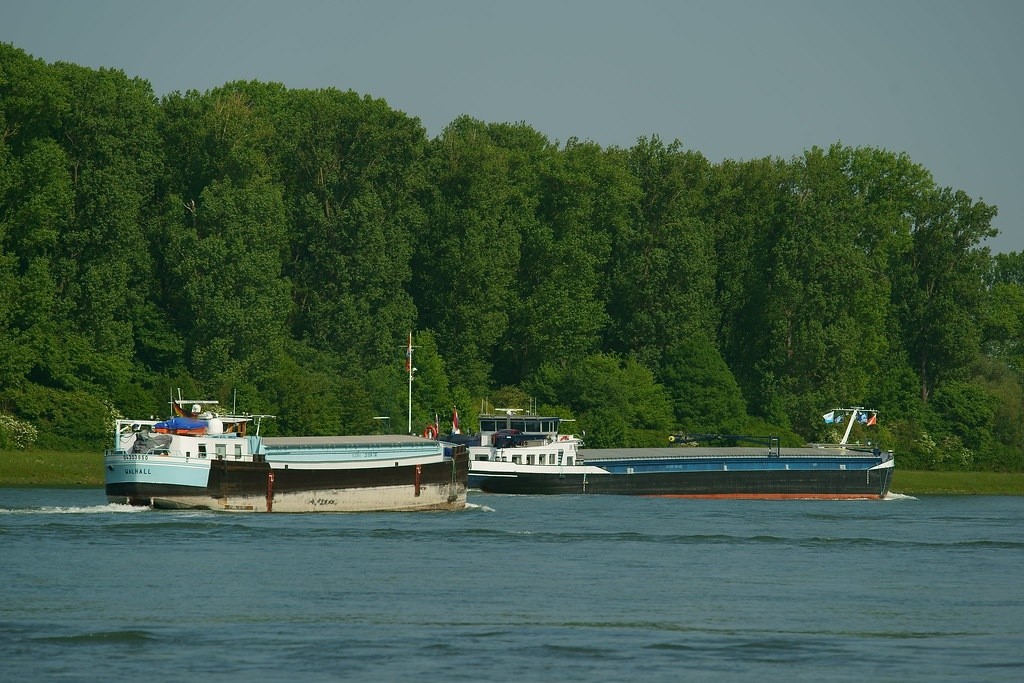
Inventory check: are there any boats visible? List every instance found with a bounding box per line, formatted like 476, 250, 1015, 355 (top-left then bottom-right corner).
424, 408, 896, 500
104, 390, 472, 513
464, 396, 611, 494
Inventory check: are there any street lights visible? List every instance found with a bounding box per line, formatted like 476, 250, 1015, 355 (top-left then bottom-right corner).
409, 368, 418, 432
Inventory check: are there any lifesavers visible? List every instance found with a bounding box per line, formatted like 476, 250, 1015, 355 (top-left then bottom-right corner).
560, 436, 569, 441
424, 426, 437, 439
